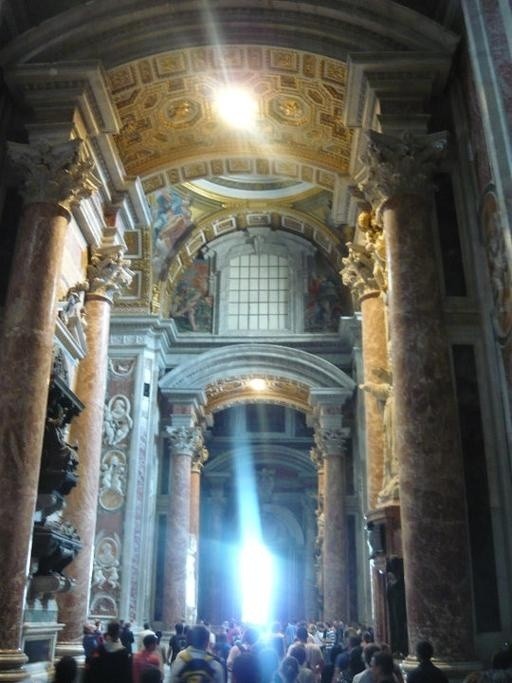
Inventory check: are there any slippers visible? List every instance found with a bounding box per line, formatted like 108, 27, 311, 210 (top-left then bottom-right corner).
177, 649, 216, 682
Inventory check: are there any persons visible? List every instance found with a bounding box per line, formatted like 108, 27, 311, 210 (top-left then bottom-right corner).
90, 394, 136, 590
